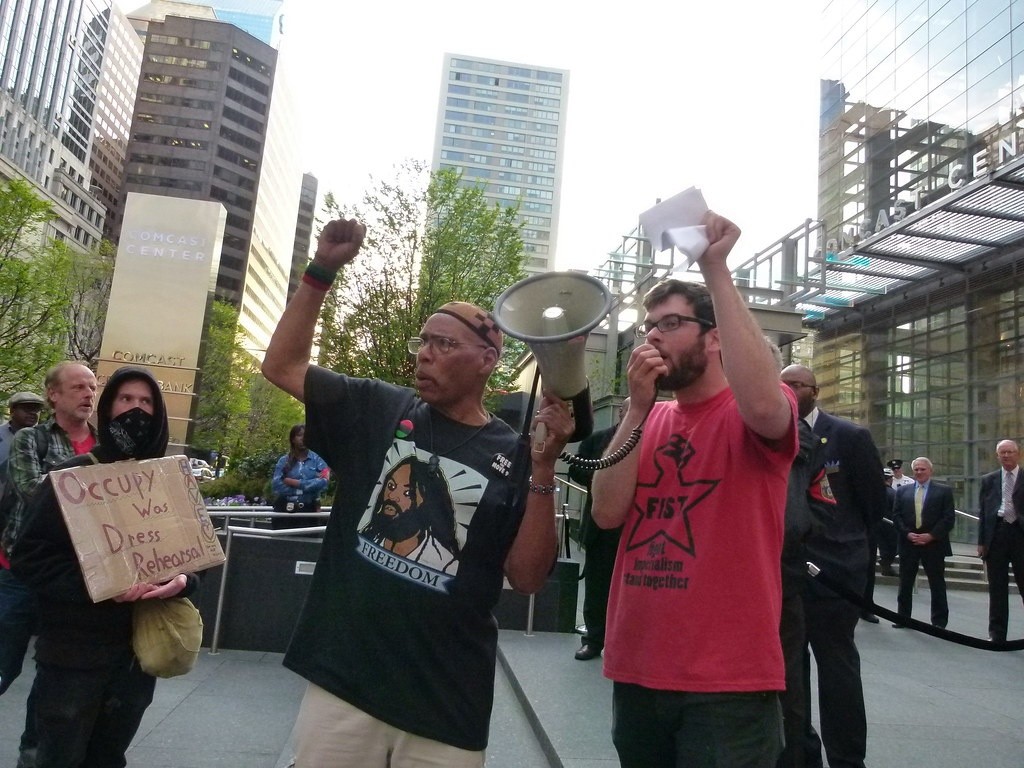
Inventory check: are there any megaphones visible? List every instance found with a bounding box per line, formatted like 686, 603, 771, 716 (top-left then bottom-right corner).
493, 270, 612, 444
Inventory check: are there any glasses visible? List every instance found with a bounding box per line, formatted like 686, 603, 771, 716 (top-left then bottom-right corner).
632, 313, 716, 339
782, 380, 815, 391
406, 336, 487, 355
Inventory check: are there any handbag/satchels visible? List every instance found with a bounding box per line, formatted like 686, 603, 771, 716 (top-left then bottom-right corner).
128, 593, 205, 680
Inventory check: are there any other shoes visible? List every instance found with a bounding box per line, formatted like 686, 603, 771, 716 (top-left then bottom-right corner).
881, 565, 900, 577
933, 624, 945, 635
861, 613, 879, 623
893, 617, 909, 628
989, 637, 1006, 650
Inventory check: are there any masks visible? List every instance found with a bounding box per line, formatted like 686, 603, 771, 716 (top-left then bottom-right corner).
106, 407, 157, 460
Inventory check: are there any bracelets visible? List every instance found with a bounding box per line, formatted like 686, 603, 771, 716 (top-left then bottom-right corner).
528, 475, 555, 495
302, 260, 337, 290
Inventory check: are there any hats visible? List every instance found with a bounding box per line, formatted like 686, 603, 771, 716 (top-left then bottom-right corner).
8, 391, 45, 408
883, 467, 895, 476
887, 459, 903, 469
433, 301, 503, 356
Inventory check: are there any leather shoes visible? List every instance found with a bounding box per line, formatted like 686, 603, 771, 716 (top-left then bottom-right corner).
575, 644, 601, 660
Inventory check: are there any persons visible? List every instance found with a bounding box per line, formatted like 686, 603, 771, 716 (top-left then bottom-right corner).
587, 211, 800, 768
567, 395, 632, 663
878, 459, 917, 577
257, 219, 576, 768
269, 422, 331, 540
890, 458, 956, 630
15, 365, 202, 768
0, 360, 101, 698
761, 363, 886, 768
976, 439, 1024, 644
858, 546, 881, 624
0, 392, 45, 535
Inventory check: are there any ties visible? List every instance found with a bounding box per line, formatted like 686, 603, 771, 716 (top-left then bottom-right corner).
1003, 471, 1018, 525
915, 483, 923, 529
